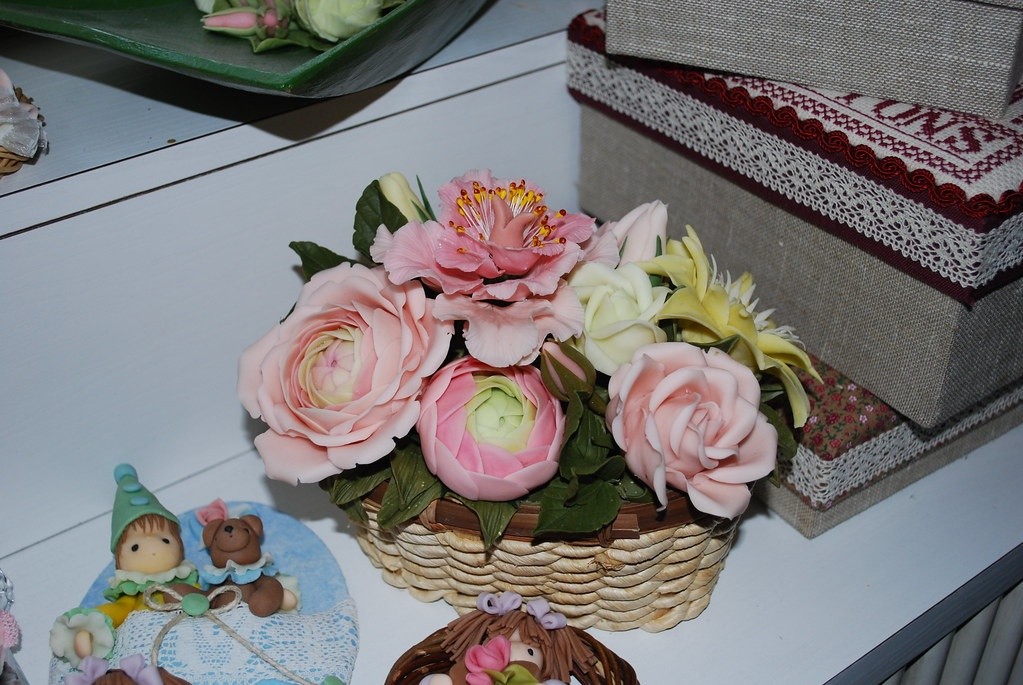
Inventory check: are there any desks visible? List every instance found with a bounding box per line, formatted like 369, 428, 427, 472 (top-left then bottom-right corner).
0, 0, 1023, 685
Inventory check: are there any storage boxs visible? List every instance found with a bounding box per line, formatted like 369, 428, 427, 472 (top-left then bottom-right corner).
751, 387, 1020, 539
563, 8, 1022, 427
605, 0, 1023, 115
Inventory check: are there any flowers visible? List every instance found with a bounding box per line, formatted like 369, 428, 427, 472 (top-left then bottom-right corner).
237, 170, 821, 543
194, 0, 407, 53
